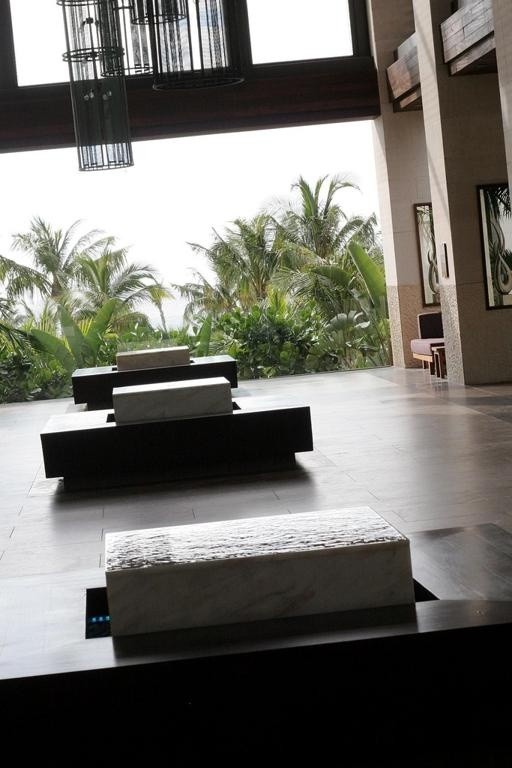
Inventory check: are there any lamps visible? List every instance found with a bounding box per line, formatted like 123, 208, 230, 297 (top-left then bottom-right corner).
58, 1, 247, 172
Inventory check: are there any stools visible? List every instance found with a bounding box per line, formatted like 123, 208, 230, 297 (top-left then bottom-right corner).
432, 345, 448, 380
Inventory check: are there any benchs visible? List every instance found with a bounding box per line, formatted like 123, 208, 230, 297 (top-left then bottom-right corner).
1, 502, 512, 768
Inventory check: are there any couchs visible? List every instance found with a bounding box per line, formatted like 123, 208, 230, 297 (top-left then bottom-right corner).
411, 311, 445, 375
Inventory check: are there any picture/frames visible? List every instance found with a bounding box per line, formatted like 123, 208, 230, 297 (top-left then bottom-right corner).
413, 202, 442, 307
475, 182, 512, 311
